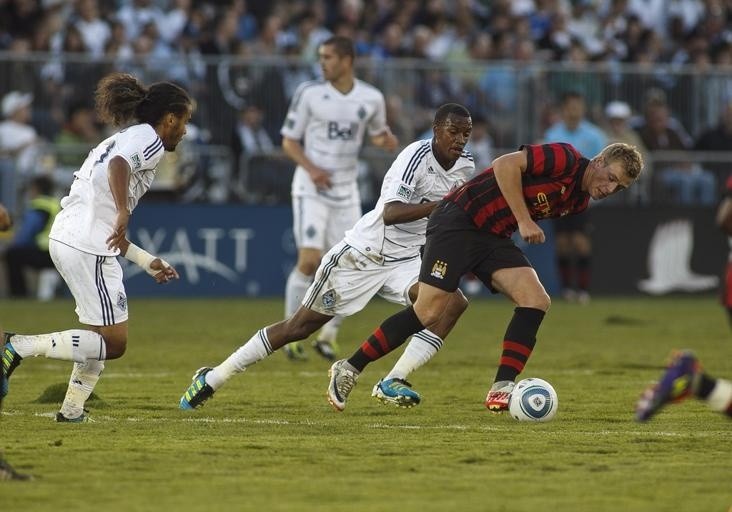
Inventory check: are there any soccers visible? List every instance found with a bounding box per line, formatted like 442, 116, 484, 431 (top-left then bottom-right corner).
508, 377, 558, 422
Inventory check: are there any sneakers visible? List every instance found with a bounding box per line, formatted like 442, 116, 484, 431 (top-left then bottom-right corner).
371, 376, 422, 409
179, 365, 217, 410
311, 332, 341, 360
0, 331, 24, 399
632, 349, 705, 423
56, 411, 102, 424
326, 359, 357, 412
285, 339, 309, 360
483, 379, 517, 412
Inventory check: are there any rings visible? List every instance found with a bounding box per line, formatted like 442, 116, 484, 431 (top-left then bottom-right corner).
115, 231, 119, 236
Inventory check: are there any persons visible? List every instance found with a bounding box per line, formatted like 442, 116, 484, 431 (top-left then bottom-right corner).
709, 164, 731, 316
1, 0, 731, 209
326, 140, 645, 413
3, 174, 64, 298
632, 341, 731, 430
279, 36, 399, 363
177, 101, 475, 411
1, 73, 201, 423
550, 208, 598, 306
